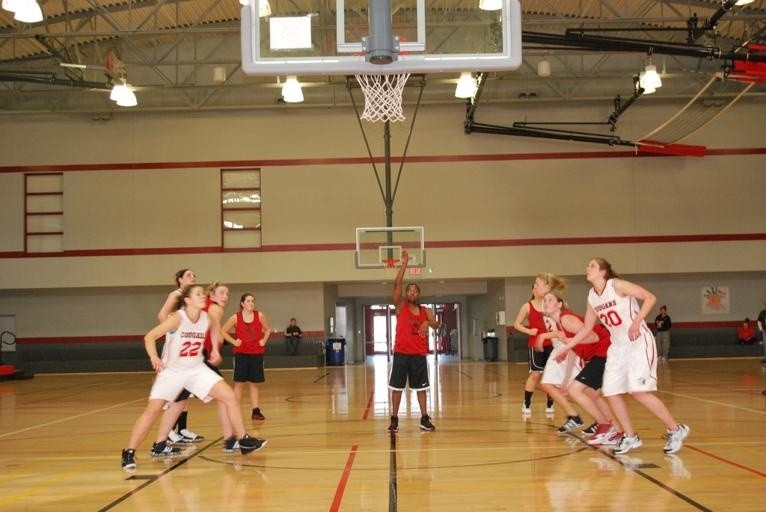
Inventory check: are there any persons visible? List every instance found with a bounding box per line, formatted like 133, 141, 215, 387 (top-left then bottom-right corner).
736, 317, 760, 346
120, 284, 269, 469
221, 293, 272, 421
653, 304, 672, 362
534, 271, 602, 435
514, 281, 556, 415
386, 249, 440, 435
536, 292, 627, 446
151, 279, 243, 457
283, 317, 303, 355
758, 307, 766, 367
160, 268, 207, 442
552, 256, 692, 456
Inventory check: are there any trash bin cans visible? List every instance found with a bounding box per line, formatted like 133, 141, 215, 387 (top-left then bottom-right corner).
482, 337, 498, 362
327, 338, 346, 366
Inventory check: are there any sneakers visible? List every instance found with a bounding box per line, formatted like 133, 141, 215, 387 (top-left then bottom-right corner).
545, 403, 554, 413
238, 433, 267, 457
251, 406, 266, 421
556, 415, 584, 435
178, 428, 206, 443
612, 432, 643, 455
387, 414, 400, 433
420, 416, 435, 432
167, 430, 184, 444
150, 440, 183, 457
120, 448, 137, 469
223, 433, 240, 453
521, 403, 531, 414
580, 422, 625, 446
663, 423, 690, 455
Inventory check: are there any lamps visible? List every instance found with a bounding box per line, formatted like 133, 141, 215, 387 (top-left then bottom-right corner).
0, 0, 43, 24
239, 0, 273, 19
477, 0, 504, 11
281, 77, 305, 103
635, 50, 662, 95
454, 68, 478, 98
108, 60, 137, 107
721, 0, 755, 6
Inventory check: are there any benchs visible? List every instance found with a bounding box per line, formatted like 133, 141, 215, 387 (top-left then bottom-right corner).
1, 337, 325, 374
505, 321, 765, 364
